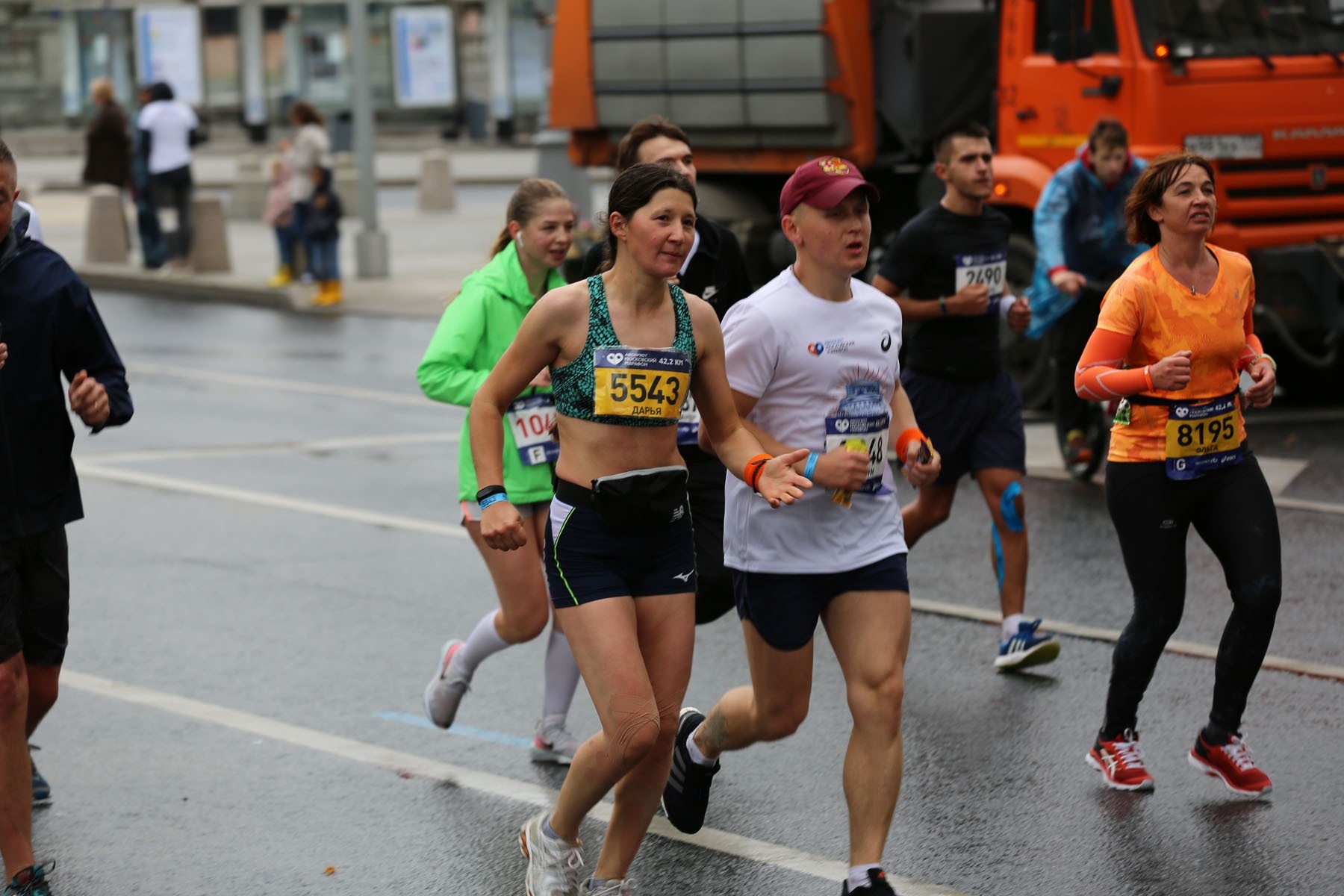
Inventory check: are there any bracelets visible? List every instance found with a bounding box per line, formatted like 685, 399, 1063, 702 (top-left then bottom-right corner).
939, 295, 950, 318
1248, 353, 1278, 371
479, 493, 508, 509
744, 453, 777, 493
999, 294, 1014, 320
1144, 363, 1155, 393
799, 450, 823, 481
895, 427, 927, 463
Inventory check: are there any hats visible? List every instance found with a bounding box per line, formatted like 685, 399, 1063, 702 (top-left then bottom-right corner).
778, 156, 881, 219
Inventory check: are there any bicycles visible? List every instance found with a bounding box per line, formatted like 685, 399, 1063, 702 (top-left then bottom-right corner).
1048, 278, 1116, 485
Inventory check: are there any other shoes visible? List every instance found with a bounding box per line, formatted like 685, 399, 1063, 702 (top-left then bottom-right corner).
1064, 429, 1091, 475
268, 263, 290, 286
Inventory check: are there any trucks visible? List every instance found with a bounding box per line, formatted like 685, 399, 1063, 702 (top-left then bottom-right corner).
547, 1, 1344, 418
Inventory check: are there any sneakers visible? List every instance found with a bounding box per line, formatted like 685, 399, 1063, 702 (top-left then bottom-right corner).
530, 724, 584, 764
1084, 726, 1155, 791
518, 808, 637, 896
660, 707, 721, 835
992, 616, 1061, 670
842, 867, 897, 896
1188, 726, 1273, 795
424, 638, 474, 730
27, 744, 53, 805
0, 858, 56, 896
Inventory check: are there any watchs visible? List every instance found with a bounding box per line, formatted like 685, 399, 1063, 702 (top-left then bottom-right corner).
476, 484, 507, 503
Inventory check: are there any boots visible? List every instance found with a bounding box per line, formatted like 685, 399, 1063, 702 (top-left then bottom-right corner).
312, 279, 343, 305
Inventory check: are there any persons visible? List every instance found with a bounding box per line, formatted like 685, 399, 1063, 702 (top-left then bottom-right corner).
418, 177, 594, 769
0, 138, 135, 896
261, 101, 347, 306
582, 114, 947, 623
469, 163, 813, 895
1074, 155, 1282, 798
663, 153, 941, 896
1019, 119, 1153, 487
874, 120, 1063, 672
81, 78, 210, 265
